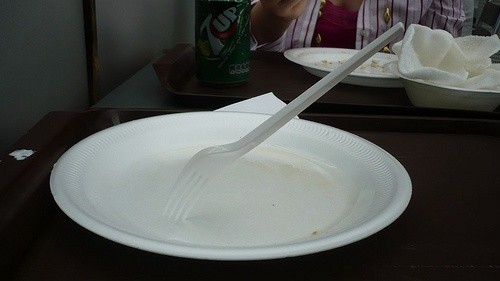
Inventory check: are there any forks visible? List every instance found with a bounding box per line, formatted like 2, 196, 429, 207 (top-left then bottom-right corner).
160, 22, 404, 222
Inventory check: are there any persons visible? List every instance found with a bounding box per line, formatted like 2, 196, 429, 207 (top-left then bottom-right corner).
250, 0, 466, 55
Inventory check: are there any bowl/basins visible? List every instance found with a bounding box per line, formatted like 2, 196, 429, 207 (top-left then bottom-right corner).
382, 61, 500, 110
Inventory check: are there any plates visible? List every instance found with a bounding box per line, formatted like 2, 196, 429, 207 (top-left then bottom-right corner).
50, 111, 412, 261
283, 47, 407, 87
